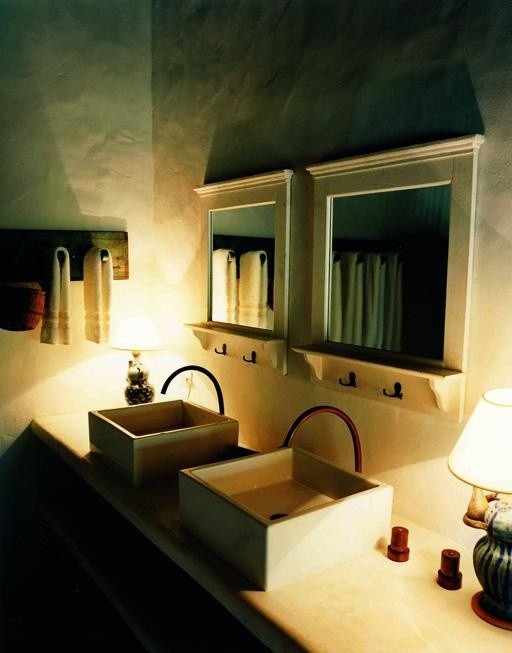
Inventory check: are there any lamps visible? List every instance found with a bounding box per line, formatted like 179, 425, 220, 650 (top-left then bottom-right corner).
445, 388, 512, 631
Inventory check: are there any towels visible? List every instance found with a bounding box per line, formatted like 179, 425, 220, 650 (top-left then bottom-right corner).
40, 243, 115, 346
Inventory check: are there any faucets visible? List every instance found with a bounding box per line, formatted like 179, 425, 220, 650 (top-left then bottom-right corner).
283, 405, 362, 472
160, 364, 224, 414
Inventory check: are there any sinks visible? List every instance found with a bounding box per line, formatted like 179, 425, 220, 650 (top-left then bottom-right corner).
178, 445, 394, 592
88, 399, 240, 488
